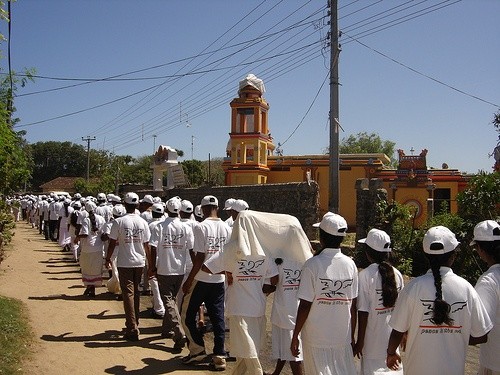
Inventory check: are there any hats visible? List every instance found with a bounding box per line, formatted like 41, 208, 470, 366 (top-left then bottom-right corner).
422, 226, 461, 254
357, 228, 392, 253
6, 192, 250, 215
312, 212, 348, 237
469, 220, 500, 246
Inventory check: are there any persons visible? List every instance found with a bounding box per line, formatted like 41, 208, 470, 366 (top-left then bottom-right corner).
202, 210, 314, 375
290, 213, 359, 375
0, 192, 73, 252
469, 219, 500, 375
387, 227, 493, 375
71, 193, 251, 371
355, 229, 408, 375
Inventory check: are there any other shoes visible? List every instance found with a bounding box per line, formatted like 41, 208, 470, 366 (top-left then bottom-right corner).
83, 288, 96, 297
183, 350, 207, 364
123, 332, 139, 342
161, 330, 175, 338
208, 354, 227, 370
146, 307, 164, 318
171, 334, 187, 354
